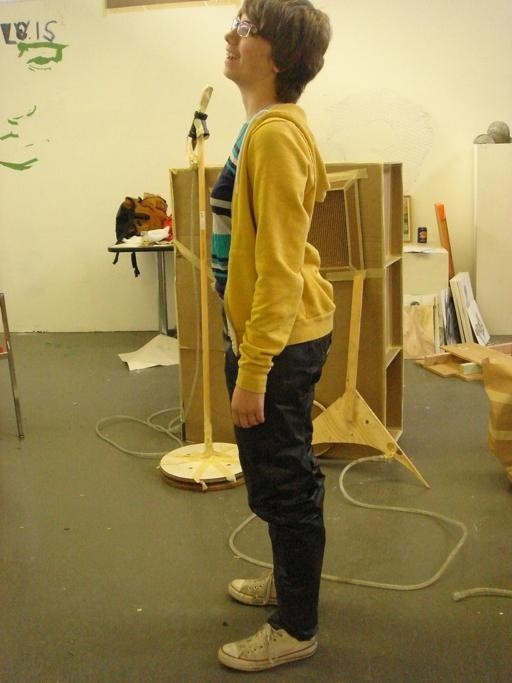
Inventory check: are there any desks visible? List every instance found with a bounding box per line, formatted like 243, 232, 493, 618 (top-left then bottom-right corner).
107, 240, 176, 336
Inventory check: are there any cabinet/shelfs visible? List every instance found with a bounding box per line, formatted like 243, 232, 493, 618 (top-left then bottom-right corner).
169, 159, 406, 460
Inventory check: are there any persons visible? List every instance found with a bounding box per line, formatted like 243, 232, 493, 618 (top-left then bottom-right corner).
210, 0, 373, 671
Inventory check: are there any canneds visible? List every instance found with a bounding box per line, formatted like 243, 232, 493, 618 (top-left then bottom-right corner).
418, 227, 427, 243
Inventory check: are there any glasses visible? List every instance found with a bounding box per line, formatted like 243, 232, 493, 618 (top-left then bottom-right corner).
231, 16, 257, 39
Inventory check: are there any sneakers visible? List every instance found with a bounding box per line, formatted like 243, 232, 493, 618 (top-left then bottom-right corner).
217, 621, 319, 674
228, 572, 280, 608
